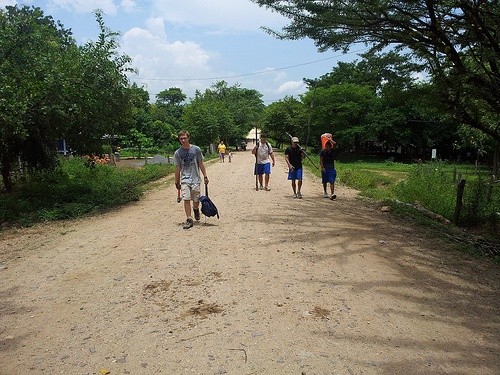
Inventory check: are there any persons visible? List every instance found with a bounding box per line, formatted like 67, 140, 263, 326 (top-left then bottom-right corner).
252, 134, 275, 191
318, 141, 339, 200
217, 140, 233, 163
174, 131, 209, 229
285, 137, 305, 199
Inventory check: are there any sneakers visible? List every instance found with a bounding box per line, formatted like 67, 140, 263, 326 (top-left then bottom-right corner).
193, 208, 200, 220
324, 193, 328, 198
183, 218, 193, 229
265, 185, 271, 191
293, 193, 302, 199
330, 194, 336, 200
260, 185, 263, 189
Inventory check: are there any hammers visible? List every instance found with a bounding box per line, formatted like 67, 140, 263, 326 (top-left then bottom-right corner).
177, 181, 182, 203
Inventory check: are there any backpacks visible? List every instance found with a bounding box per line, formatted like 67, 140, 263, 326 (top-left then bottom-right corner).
199, 183, 219, 219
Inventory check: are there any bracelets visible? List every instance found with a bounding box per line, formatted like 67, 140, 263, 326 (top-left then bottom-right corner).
204, 176, 207, 179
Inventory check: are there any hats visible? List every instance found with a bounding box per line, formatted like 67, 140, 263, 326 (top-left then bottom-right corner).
292, 137, 299, 143
